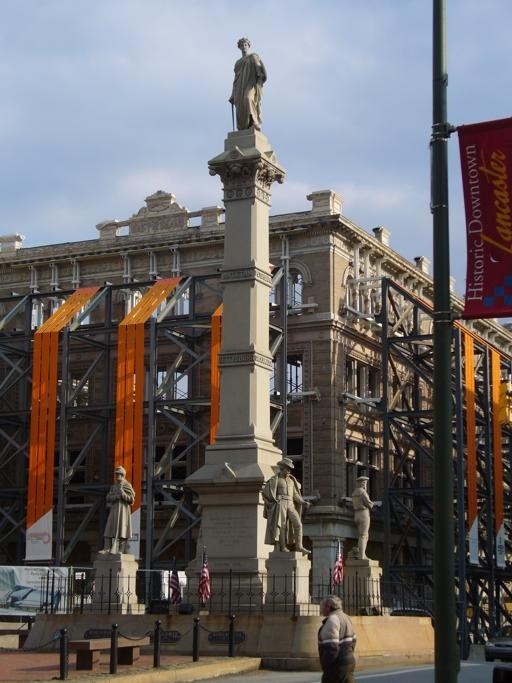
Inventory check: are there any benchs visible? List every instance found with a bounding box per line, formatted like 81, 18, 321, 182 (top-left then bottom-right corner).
68, 635, 151, 671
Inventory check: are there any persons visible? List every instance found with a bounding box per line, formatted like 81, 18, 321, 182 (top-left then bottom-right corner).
98, 466, 135, 554
317, 595, 356, 683
229, 38, 267, 131
352, 476, 374, 560
262, 458, 312, 555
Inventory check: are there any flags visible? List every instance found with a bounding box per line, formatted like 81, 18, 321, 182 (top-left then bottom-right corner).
199, 559, 215, 607
331, 542, 344, 590
168, 559, 182, 604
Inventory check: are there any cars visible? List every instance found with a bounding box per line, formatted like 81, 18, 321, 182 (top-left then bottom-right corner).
388, 607, 433, 616
483, 625, 512, 662
